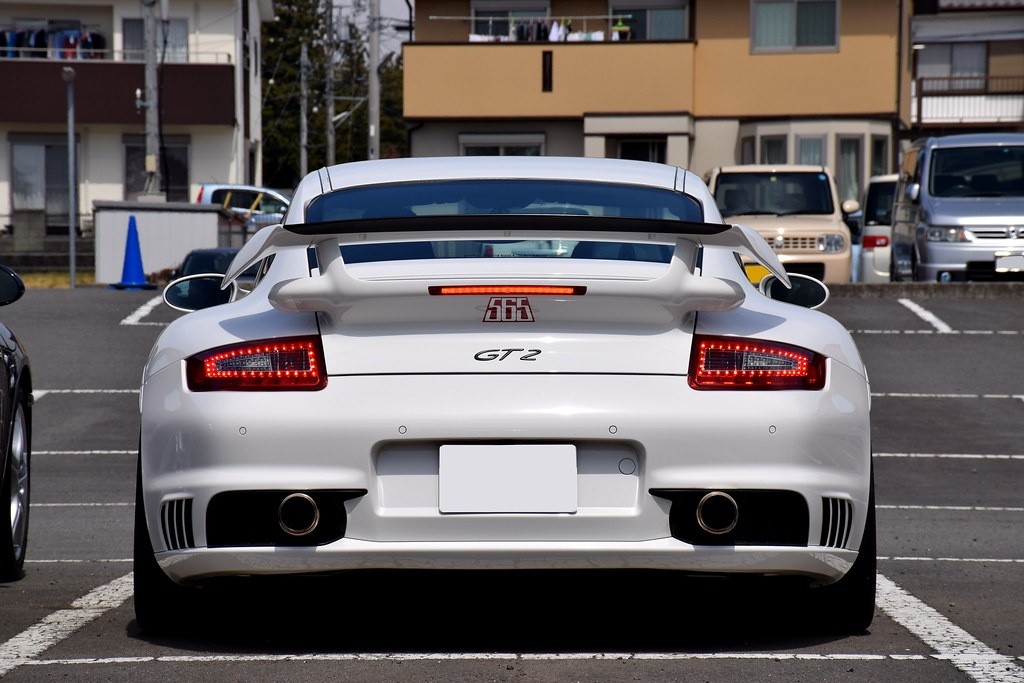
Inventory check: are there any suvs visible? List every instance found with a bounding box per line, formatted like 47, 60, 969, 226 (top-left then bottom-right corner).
707, 164, 860, 286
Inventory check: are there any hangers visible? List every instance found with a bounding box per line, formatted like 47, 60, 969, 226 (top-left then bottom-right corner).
512, 15, 573, 26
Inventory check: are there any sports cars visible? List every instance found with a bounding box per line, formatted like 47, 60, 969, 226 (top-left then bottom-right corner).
134, 154, 875, 635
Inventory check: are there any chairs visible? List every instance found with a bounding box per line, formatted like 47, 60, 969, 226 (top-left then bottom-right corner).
570, 241, 670, 262
938, 165, 970, 191
351, 206, 436, 261
724, 189, 752, 212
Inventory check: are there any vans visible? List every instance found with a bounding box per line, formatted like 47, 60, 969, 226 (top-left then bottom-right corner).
861, 133, 1024, 282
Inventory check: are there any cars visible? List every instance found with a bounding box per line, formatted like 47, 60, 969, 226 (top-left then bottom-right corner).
197, 180, 293, 227
